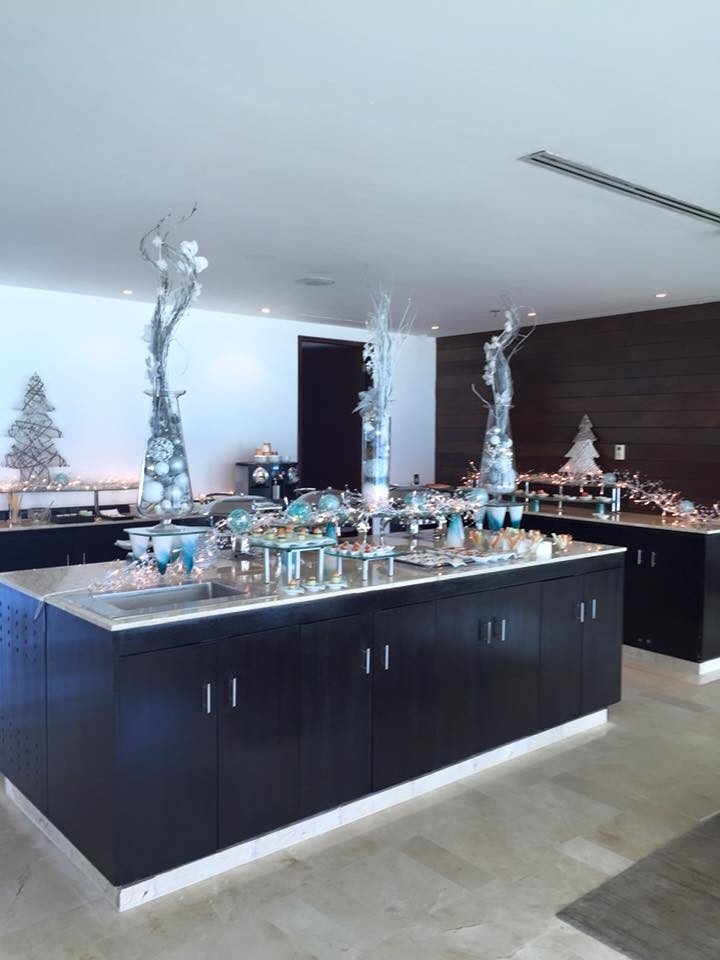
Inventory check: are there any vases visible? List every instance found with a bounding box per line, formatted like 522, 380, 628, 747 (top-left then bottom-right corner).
361, 416, 391, 501
480, 405, 516, 492
136, 389, 194, 518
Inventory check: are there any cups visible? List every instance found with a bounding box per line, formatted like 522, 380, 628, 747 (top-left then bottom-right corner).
470, 529, 540, 552
256, 443, 278, 455
553, 535, 568, 554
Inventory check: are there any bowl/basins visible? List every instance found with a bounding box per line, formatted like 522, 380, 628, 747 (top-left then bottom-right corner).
254, 455, 290, 463
27, 507, 52, 525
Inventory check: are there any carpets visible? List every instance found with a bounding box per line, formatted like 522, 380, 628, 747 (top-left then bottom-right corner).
556, 813, 720, 960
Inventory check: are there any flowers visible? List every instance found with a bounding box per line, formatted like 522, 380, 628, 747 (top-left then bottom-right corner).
138, 203, 209, 389
351, 281, 424, 415
474, 294, 538, 404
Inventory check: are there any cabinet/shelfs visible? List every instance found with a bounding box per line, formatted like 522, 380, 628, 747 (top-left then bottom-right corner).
0, 461, 720, 913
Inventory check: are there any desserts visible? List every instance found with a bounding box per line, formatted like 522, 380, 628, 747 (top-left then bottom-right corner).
332, 541, 379, 553
288, 572, 341, 588
253, 528, 323, 543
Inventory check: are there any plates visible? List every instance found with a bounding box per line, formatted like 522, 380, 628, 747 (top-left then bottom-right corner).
592, 513, 610, 519
281, 579, 348, 594
